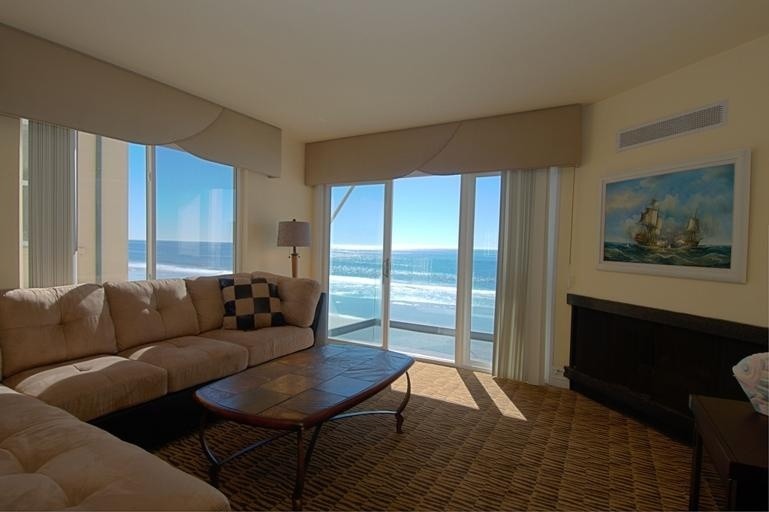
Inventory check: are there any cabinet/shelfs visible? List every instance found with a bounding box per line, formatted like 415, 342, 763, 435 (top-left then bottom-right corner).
559, 292, 768, 449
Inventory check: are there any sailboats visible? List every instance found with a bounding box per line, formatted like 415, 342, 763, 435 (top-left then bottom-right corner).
628, 196, 703, 256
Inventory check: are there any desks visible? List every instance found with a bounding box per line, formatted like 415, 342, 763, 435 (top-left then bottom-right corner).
192, 343, 415, 512
688, 392, 768, 512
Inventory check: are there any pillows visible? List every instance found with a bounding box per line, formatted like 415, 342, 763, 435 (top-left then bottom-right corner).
218, 277, 287, 332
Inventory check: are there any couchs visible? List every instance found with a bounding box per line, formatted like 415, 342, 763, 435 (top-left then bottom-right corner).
0, 381, 231, 512
0, 271, 327, 452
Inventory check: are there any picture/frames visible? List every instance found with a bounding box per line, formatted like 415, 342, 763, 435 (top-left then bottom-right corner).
594, 148, 753, 285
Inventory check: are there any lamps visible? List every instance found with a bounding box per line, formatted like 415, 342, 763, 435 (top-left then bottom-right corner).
276, 219, 311, 279
